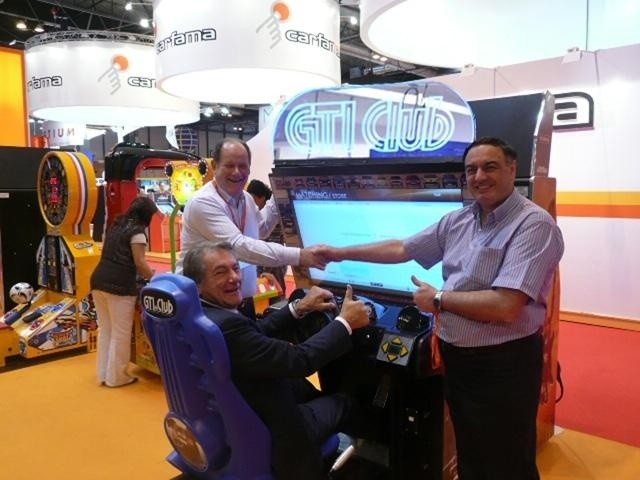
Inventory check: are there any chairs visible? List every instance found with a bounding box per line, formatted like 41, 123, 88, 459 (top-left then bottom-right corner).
140, 272, 363, 479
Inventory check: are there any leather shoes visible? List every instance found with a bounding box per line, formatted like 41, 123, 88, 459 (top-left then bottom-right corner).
128, 377, 138, 384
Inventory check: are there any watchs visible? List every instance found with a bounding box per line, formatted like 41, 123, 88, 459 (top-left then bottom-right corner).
433, 290, 446, 314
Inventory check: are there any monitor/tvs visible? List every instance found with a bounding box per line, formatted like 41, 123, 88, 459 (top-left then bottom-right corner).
269, 172, 530, 304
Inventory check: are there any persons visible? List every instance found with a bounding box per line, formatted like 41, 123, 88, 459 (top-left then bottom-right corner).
181, 241, 393, 477
88, 196, 157, 389
311, 136, 565, 480
247, 178, 272, 210
174, 138, 328, 322
